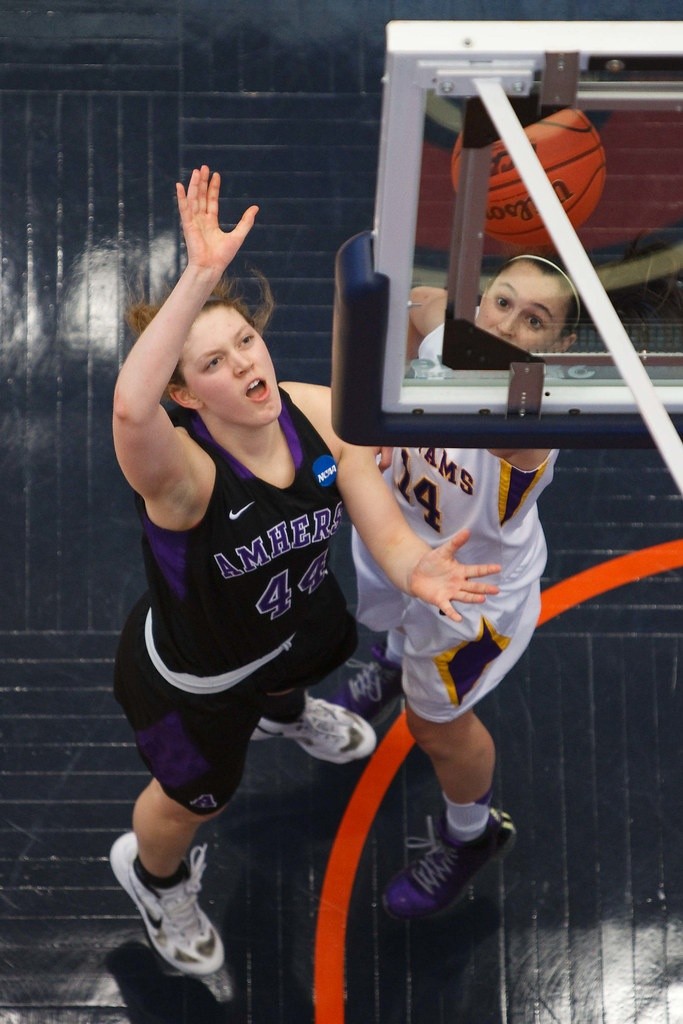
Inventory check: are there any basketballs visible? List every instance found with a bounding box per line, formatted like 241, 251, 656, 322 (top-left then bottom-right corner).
452, 103, 607, 244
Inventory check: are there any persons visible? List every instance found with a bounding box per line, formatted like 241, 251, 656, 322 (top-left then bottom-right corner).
333, 250, 585, 926
109, 162, 503, 976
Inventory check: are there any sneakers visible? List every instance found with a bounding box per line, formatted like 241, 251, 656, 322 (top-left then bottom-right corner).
383, 809, 517, 923
249, 688, 377, 764
328, 645, 403, 724
110, 830, 224, 975
